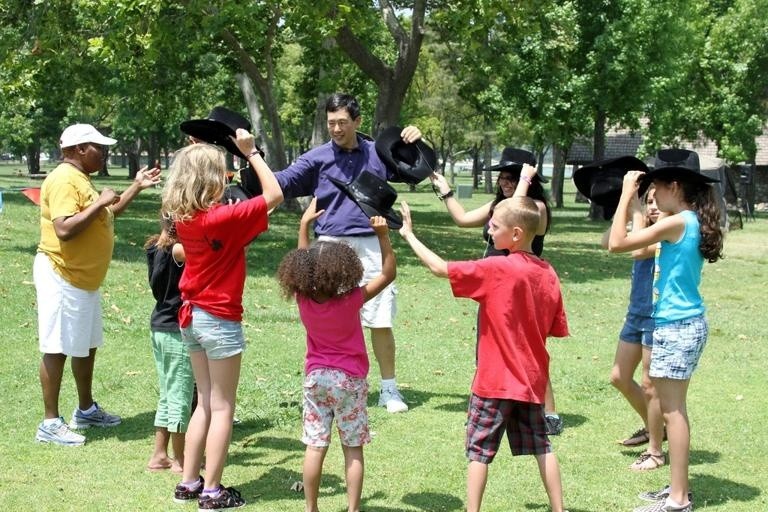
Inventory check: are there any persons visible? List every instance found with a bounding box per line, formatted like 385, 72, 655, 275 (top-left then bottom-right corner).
606, 147, 726, 512
600, 154, 667, 472
399, 195, 570, 512
430, 145, 564, 437
143, 205, 208, 476
31, 123, 164, 449
271, 193, 398, 512
164, 125, 285, 512
265, 90, 424, 415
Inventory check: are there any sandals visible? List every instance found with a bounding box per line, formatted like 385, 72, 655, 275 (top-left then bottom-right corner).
625, 428, 667, 446
630, 452, 666, 470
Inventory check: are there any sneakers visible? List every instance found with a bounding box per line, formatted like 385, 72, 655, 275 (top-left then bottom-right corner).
69, 407, 122, 428
545, 415, 560, 436
37, 420, 86, 446
638, 486, 692, 503
198, 488, 246, 511
634, 500, 693, 512
378, 390, 409, 413
174, 479, 224, 500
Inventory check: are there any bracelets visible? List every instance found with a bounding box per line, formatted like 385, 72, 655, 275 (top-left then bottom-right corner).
246, 150, 261, 158
520, 174, 532, 186
437, 190, 453, 202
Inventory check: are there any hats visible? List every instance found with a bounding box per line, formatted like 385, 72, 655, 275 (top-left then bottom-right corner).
325, 172, 403, 230
640, 149, 723, 185
374, 126, 436, 183
481, 148, 551, 185
573, 156, 650, 219
60, 124, 118, 149
218, 184, 251, 204
181, 108, 265, 161
